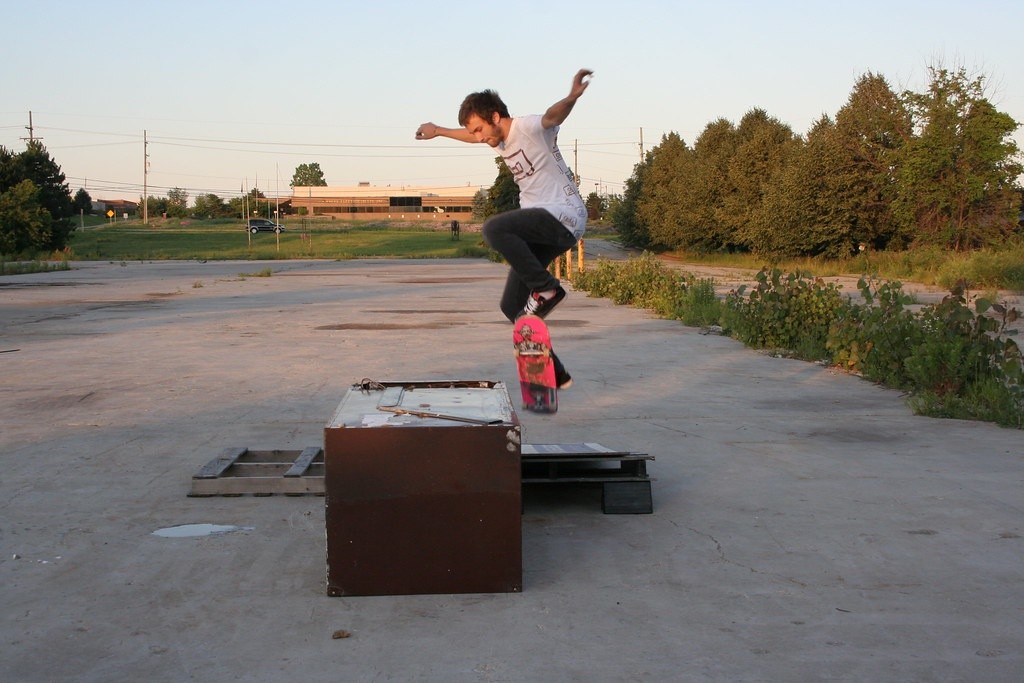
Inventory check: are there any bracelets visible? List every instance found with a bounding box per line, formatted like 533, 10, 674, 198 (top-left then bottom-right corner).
433, 126, 440, 136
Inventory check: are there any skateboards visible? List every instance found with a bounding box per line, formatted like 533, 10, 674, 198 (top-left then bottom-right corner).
512, 314, 559, 415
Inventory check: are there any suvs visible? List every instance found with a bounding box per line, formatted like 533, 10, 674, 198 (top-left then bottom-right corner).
246, 219, 285, 235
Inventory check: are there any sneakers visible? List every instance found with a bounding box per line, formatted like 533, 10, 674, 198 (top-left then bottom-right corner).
515, 285, 566, 323
556, 372, 573, 388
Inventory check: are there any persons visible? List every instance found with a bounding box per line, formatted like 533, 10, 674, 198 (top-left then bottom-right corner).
416, 70, 592, 391
